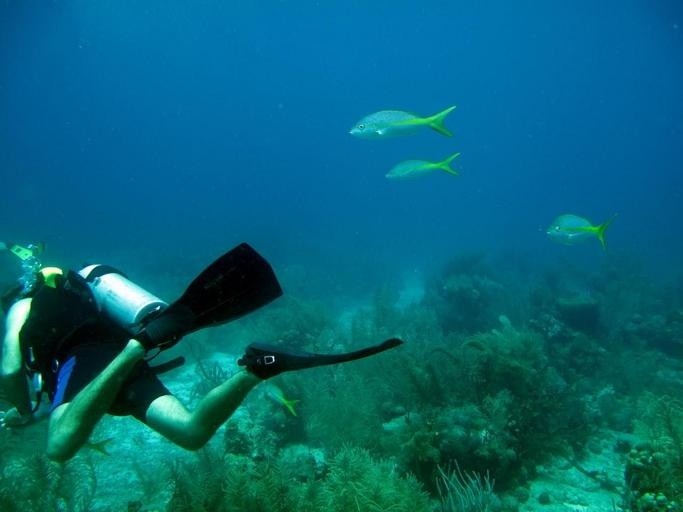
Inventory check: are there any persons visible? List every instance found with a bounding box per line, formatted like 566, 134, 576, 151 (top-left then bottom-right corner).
0, 242, 404, 461
